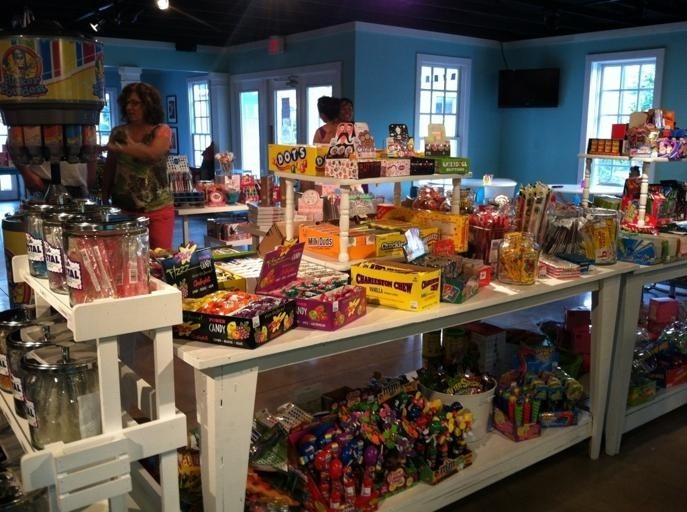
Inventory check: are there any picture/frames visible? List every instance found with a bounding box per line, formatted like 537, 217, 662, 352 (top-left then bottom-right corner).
165, 96, 178, 155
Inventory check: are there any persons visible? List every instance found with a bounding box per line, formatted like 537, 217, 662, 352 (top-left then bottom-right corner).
101, 82, 177, 250
312, 95, 355, 145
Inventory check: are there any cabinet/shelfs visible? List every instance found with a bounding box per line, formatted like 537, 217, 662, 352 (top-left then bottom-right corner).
0, 166, 686, 512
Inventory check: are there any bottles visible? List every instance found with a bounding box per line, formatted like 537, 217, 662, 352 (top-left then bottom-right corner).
58, 204, 152, 308
21, 194, 75, 279
495, 230, 542, 286
572, 206, 621, 265
39, 195, 102, 295
0, 308, 102, 452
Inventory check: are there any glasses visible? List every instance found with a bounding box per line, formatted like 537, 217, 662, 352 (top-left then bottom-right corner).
124, 100, 143, 105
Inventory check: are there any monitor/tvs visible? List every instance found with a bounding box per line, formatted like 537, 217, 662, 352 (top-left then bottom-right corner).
498, 67, 560, 108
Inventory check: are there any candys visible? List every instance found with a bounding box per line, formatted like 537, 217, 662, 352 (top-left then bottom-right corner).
31, 217, 151, 304
